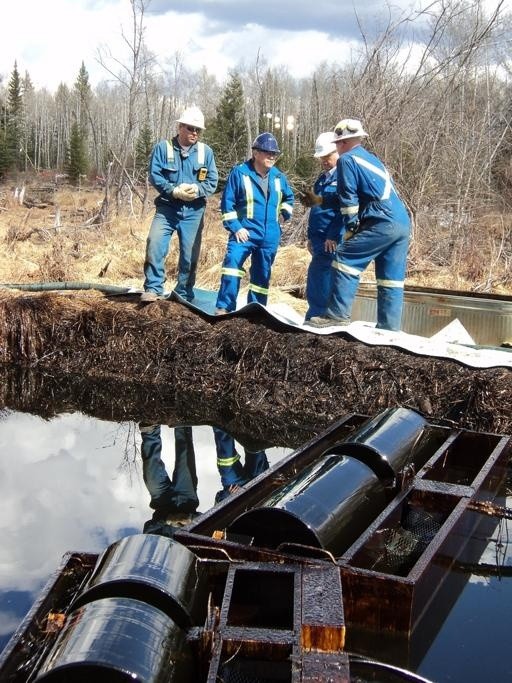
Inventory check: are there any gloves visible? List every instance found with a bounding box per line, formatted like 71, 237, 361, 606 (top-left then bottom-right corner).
185, 184, 199, 195
173, 183, 196, 203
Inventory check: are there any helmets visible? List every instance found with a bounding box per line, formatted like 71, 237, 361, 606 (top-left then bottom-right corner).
331, 118, 370, 145
313, 130, 337, 158
175, 105, 208, 134
250, 130, 281, 155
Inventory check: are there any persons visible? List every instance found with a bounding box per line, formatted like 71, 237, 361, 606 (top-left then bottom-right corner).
141, 105, 219, 303
301, 118, 411, 330
136, 420, 205, 535
303, 131, 345, 323
215, 130, 294, 314
211, 425, 272, 507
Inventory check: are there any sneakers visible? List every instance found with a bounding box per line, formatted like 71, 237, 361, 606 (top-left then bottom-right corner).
307, 311, 351, 329
214, 304, 232, 316
138, 421, 158, 434
139, 288, 164, 304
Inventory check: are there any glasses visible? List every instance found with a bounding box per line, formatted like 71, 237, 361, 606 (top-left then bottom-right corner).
182, 123, 202, 133
